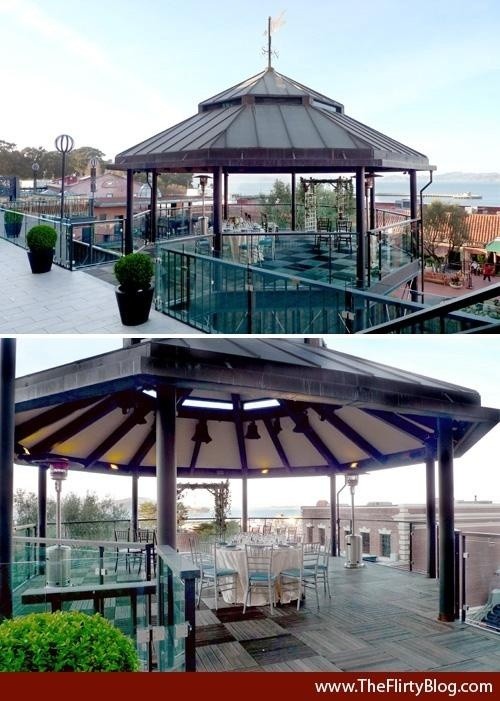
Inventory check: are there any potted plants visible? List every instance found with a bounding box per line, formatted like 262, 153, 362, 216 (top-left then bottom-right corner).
25, 225, 57, 274
4, 208, 23, 238
113, 253, 155, 326
449, 273, 463, 289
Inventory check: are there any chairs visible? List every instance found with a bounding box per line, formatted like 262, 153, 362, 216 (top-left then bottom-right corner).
113, 526, 334, 616
314, 216, 353, 254
195, 212, 279, 266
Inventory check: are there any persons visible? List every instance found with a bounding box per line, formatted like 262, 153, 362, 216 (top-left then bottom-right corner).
482, 263, 493, 283
469, 261, 482, 276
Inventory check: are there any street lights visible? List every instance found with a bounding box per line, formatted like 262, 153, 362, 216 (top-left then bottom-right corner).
32, 163, 40, 193
54, 135, 74, 222
91, 159, 98, 198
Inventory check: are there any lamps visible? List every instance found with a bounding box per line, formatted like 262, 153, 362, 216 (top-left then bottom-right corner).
109, 389, 337, 444
